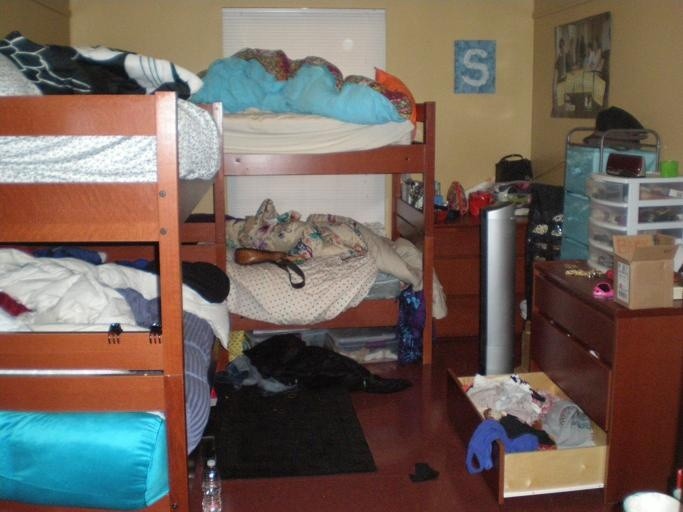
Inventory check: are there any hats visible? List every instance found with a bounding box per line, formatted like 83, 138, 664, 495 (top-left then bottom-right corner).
582, 105, 648, 148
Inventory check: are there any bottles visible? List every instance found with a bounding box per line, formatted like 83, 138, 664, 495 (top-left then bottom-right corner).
202, 459, 222, 512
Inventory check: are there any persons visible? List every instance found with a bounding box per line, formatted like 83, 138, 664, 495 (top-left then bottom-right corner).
553, 33, 604, 113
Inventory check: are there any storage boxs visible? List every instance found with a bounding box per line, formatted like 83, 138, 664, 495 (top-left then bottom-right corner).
610, 229, 681, 311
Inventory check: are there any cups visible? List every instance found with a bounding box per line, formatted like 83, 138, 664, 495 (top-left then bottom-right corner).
468, 191, 490, 216
660, 160, 679, 178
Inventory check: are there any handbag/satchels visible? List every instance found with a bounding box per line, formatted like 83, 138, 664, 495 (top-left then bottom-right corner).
234, 245, 287, 266
494, 153, 533, 183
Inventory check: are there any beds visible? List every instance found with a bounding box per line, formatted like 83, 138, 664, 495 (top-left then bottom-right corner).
213, 101, 435, 376
0, 91, 223, 512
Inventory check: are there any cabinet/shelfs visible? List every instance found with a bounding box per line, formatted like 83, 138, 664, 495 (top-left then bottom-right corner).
431, 209, 533, 343
560, 126, 683, 281
445, 260, 683, 512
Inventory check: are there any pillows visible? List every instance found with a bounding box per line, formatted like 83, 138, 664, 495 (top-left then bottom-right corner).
373, 66, 419, 127
